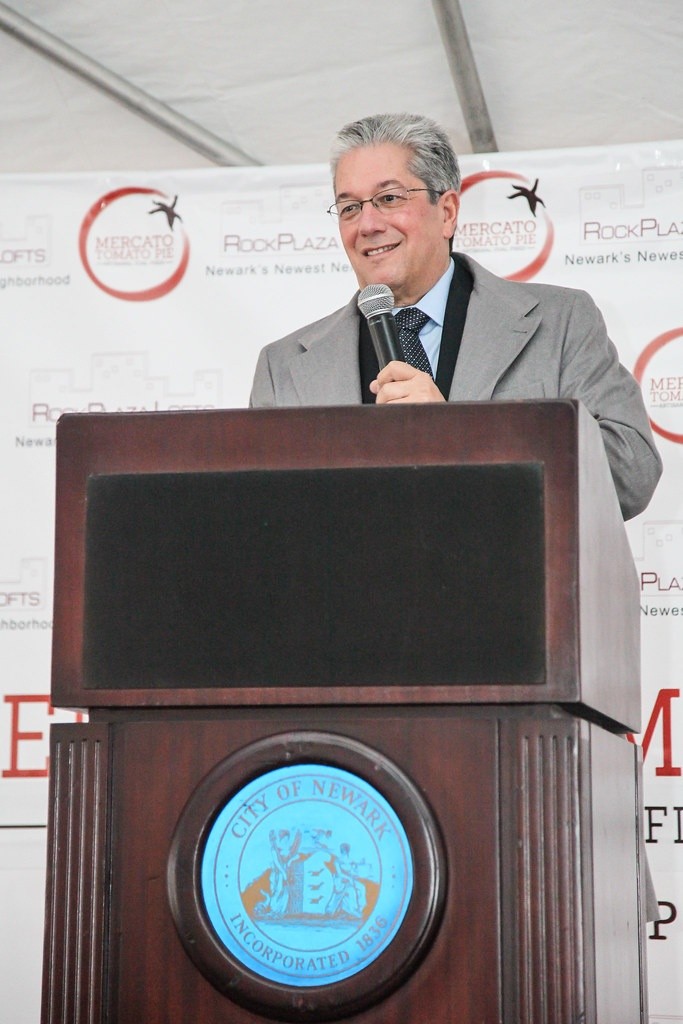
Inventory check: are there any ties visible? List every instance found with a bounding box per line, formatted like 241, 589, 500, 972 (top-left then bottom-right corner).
391, 307, 435, 384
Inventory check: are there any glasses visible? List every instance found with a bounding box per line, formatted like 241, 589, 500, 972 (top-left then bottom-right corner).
326, 184, 446, 223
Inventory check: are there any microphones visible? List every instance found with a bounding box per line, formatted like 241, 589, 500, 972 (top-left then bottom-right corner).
357, 284, 407, 371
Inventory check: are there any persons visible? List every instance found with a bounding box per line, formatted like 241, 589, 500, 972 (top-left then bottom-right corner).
246, 113, 663, 921
